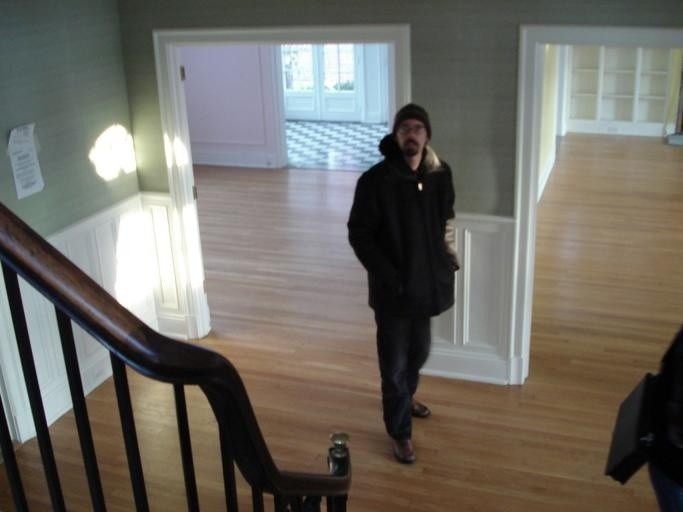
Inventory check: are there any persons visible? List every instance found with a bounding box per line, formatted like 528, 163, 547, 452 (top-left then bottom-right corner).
343, 105, 459, 465
643, 318, 682, 509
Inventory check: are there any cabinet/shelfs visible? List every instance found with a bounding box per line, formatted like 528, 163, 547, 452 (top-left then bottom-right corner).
561, 42, 676, 138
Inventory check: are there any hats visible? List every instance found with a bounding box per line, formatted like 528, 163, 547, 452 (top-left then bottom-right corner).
392, 103, 430, 140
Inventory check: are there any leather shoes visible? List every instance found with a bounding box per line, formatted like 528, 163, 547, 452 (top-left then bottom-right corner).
391, 438, 415, 463
410, 399, 430, 418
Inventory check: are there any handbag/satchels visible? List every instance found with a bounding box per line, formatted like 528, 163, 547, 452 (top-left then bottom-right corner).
604, 373, 655, 484
